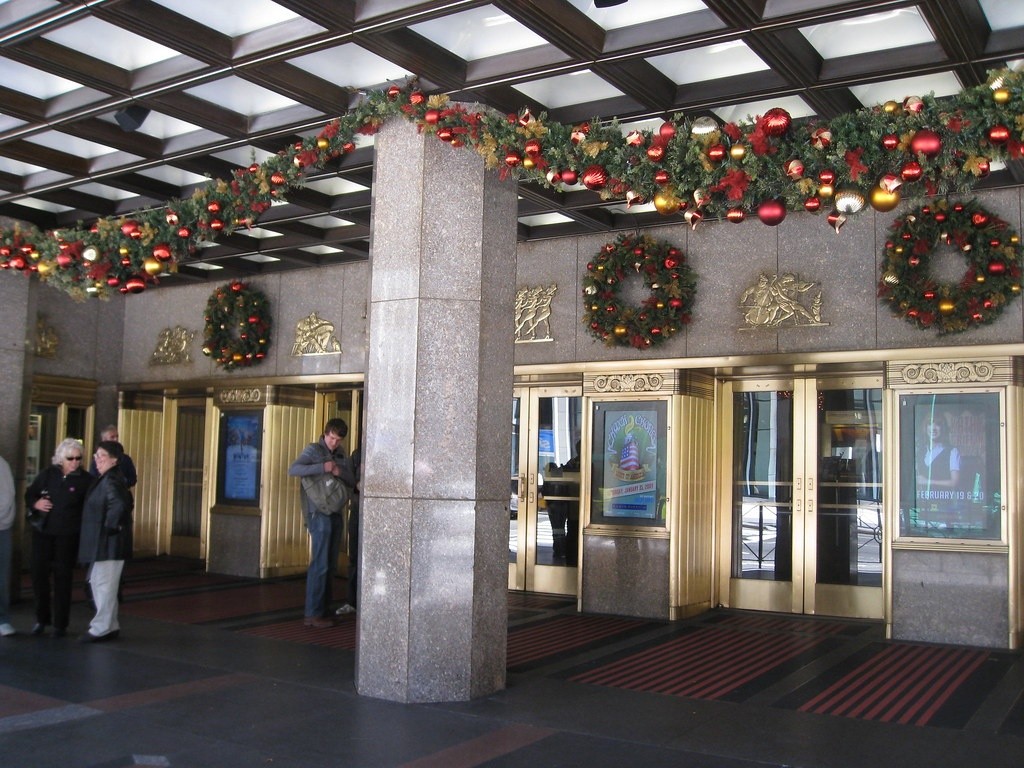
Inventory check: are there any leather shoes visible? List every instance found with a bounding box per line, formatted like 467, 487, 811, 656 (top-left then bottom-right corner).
326, 612, 344, 623
304, 616, 337, 629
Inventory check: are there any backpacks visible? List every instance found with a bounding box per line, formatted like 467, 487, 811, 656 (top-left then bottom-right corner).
300, 443, 350, 516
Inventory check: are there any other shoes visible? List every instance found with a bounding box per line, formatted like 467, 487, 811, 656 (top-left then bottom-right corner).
0, 623, 15, 636
32, 623, 44, 635
51, 628, 66, 638
77, 629, 119, 643
336, 604, 355, 615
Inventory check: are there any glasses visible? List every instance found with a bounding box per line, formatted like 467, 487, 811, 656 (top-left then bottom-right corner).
65, 456, 83, 461
93, 452, 109, 460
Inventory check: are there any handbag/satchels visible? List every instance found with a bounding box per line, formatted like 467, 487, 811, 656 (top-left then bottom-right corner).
25, 466, 54, 533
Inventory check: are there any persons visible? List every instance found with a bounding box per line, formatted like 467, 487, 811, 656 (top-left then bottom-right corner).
915, 413, 961, 531
90, 426, 118, 474
77, 441, 137, 641
0, 456, 17, 635
24, 438, 96, 640
289, 418, 362, 627
541, 440, 594, 566
